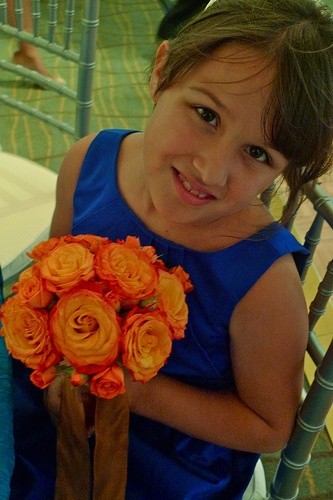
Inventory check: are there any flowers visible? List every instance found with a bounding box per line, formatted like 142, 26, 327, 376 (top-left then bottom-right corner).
1, 234, 194, 400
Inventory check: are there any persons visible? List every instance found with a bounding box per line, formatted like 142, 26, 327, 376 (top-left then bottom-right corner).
9, 0, 332, 500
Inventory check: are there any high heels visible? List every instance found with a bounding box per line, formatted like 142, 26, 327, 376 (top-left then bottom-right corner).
14, 50, 66, 89
